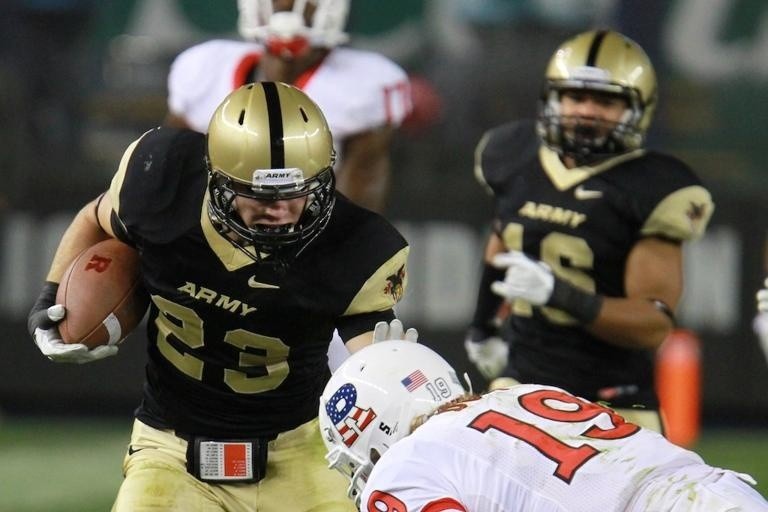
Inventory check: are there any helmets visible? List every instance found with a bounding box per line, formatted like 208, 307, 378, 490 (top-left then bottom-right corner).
205, 80, 335, 195
545, 28, 658, 151
317, 340, 467, 480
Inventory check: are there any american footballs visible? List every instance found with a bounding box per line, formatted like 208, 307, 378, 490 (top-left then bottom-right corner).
53, 238, 152, 350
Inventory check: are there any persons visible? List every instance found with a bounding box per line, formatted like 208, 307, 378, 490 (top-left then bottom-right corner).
158, 0, 415, 211
755, 277, 768, 313
313, 332, 767, 511
25, 75, 423, 512
460, 29, 715, 447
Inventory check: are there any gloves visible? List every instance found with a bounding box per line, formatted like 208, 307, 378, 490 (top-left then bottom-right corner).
489, 250, 556, 305
464, 316, 509, 381
373, 318, 420, 344
25, 280, 120, 366
